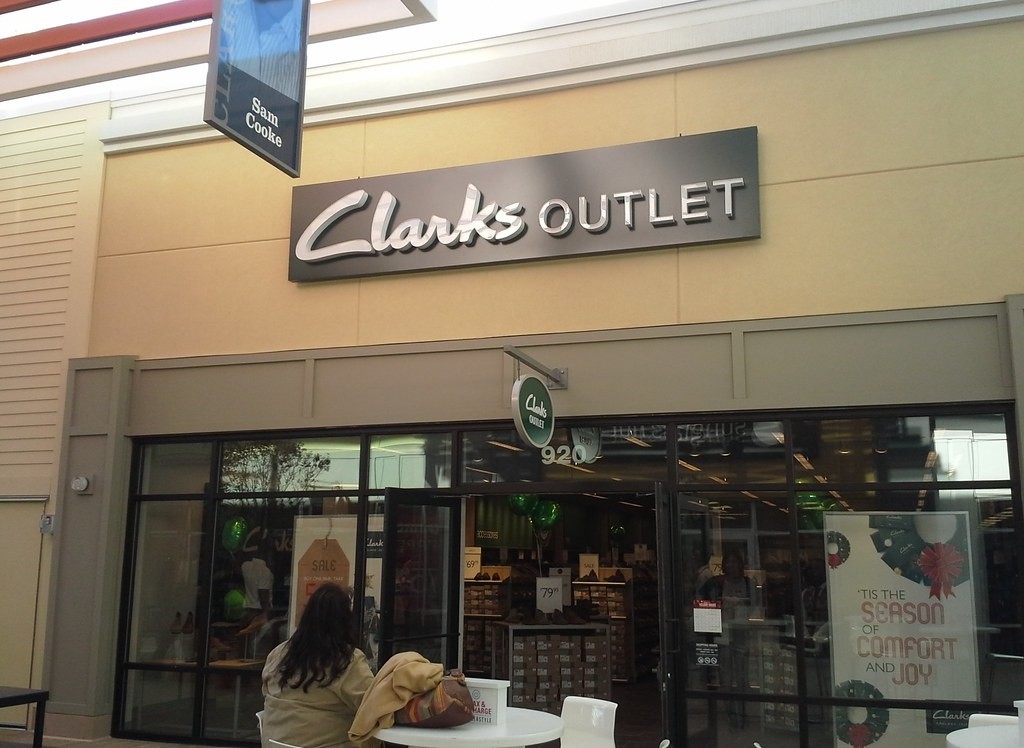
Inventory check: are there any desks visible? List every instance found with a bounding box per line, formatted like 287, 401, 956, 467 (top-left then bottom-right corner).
724, 618, 827, 733
491, 620, 611, 708
0, 685, 50, 748
945, 725, 1020, 747
373, 707, 562, 748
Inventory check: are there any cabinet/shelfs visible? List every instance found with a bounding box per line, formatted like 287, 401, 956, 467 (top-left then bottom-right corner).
463, 579, 631, 683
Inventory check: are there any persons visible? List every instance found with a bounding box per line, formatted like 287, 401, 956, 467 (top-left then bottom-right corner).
701, 549, 761, 731
693, 551, 717, 645
241, 544, 279, 659
262, 582, 376, 748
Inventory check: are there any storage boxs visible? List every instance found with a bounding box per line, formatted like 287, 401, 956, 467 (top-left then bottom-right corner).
464, 583, 627, 679
731, 640, 822, 733
505, 636, 609, 717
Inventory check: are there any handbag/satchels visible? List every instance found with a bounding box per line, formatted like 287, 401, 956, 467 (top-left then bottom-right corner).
393, 669, 475, 728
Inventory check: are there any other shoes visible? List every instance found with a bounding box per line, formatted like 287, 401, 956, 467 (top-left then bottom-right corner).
474, 573, 490, 582
493, 573, 500, 580
577, 599, 599, 608
582, 569, 599, 582
507, 608, 590, 626
607, 569, 625, 582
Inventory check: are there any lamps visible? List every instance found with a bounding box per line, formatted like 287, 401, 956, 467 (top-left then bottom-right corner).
327, 433, 936, 513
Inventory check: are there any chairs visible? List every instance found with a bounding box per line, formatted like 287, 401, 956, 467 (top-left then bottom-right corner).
560, 695, 619, 748
967, 713, 1018, 728
